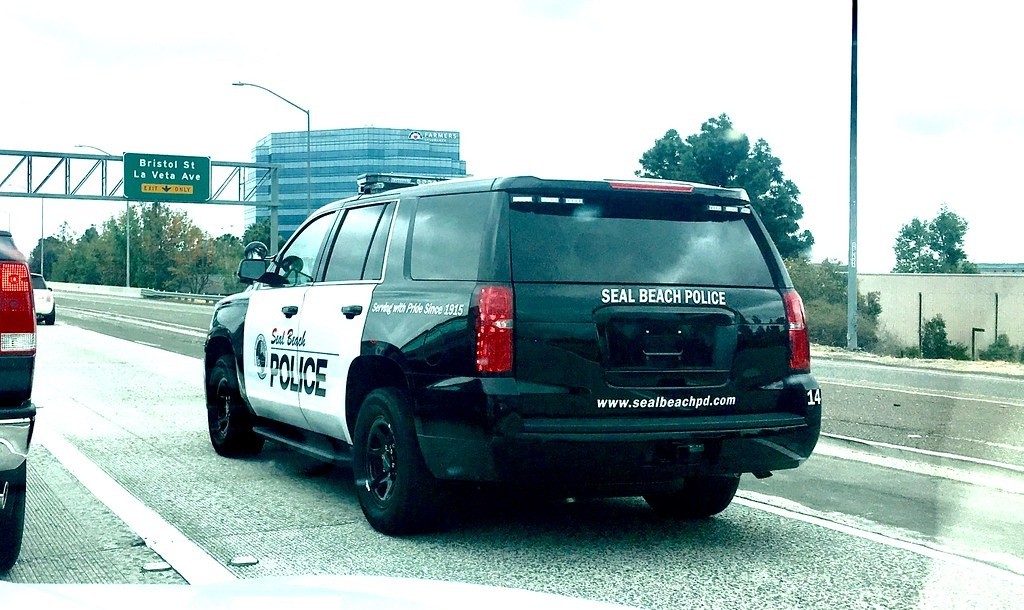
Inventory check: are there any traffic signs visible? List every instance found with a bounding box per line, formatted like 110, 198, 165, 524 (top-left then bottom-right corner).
122, 153, 212, 204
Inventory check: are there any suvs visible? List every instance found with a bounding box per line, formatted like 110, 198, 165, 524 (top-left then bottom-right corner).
203, 171, 822, 535
0, 230, 37, 569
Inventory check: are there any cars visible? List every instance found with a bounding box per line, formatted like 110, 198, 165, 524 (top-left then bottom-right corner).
28, 273, 55, 325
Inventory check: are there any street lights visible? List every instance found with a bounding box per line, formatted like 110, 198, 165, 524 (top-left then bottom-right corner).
233, 82, 311, 219
74, 144, 130, 287
8, 184, 44, 277
